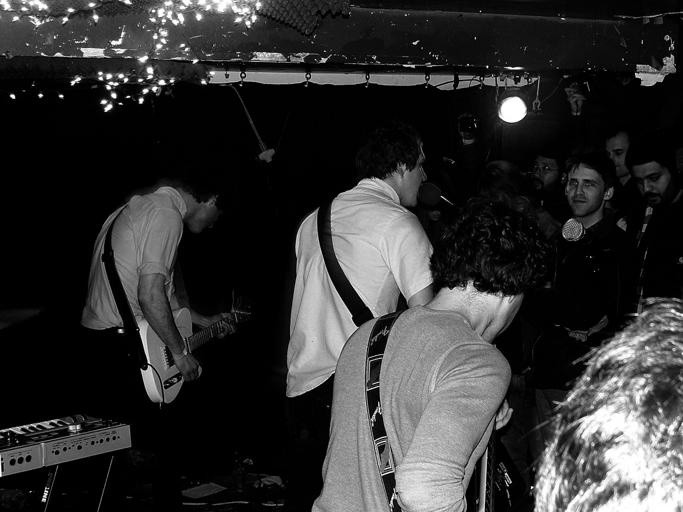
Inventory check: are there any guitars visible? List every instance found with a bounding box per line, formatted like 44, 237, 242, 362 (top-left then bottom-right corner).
138, 288, 252, 403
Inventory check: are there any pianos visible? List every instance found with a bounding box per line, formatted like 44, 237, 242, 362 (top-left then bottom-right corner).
0, 414, 134, 479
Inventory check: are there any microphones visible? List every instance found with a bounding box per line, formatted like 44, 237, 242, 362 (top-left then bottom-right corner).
561, 218, 616, 259
419, 183, 456, 206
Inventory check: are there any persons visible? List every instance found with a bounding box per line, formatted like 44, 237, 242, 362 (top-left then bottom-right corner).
479, 118, 683, 511
281, 116, 451, 512
307, 193, 550, 511
75, 155, 242, 511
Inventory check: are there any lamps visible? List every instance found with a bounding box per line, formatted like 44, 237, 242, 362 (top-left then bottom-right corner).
494, 85, 531, 125
